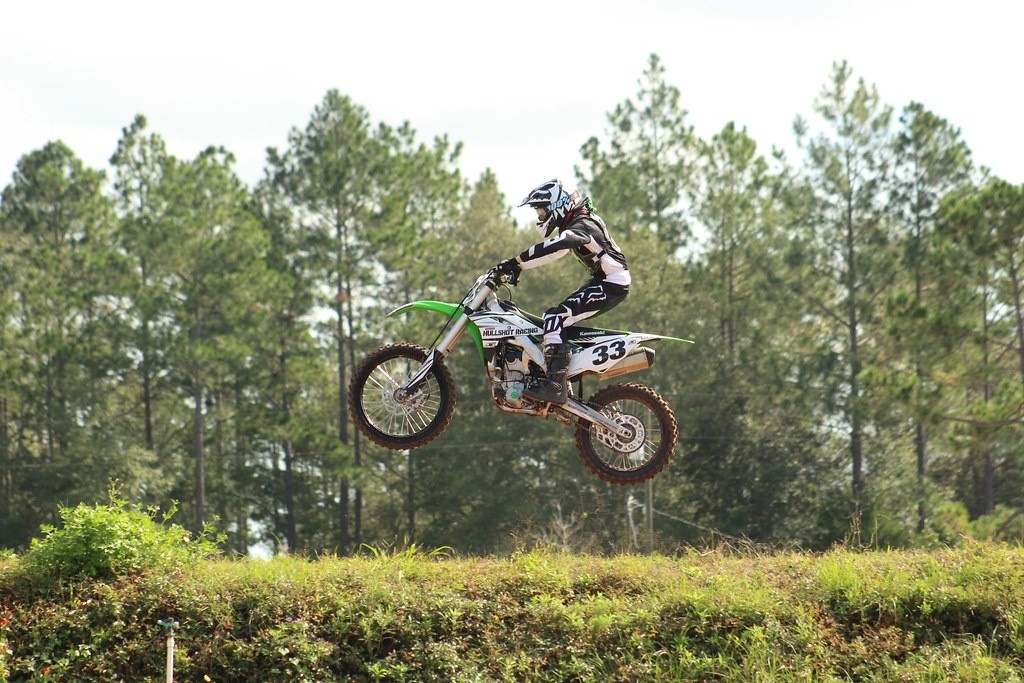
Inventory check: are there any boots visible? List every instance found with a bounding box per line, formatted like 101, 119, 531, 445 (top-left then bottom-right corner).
521, 343, 572, 404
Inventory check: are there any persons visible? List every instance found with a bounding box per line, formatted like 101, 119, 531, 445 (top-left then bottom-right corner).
486, 170, 631, 404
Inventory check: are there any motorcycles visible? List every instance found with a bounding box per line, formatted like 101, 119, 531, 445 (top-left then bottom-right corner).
347, 262, 695, 489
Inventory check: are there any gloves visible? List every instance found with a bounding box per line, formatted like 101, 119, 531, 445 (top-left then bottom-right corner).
493, 257, 522, 287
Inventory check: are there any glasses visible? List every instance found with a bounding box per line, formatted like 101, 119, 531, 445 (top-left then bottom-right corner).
535, 207, 548, 221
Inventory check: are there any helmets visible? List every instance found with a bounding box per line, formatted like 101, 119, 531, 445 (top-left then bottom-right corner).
518, 179, 574, 239
